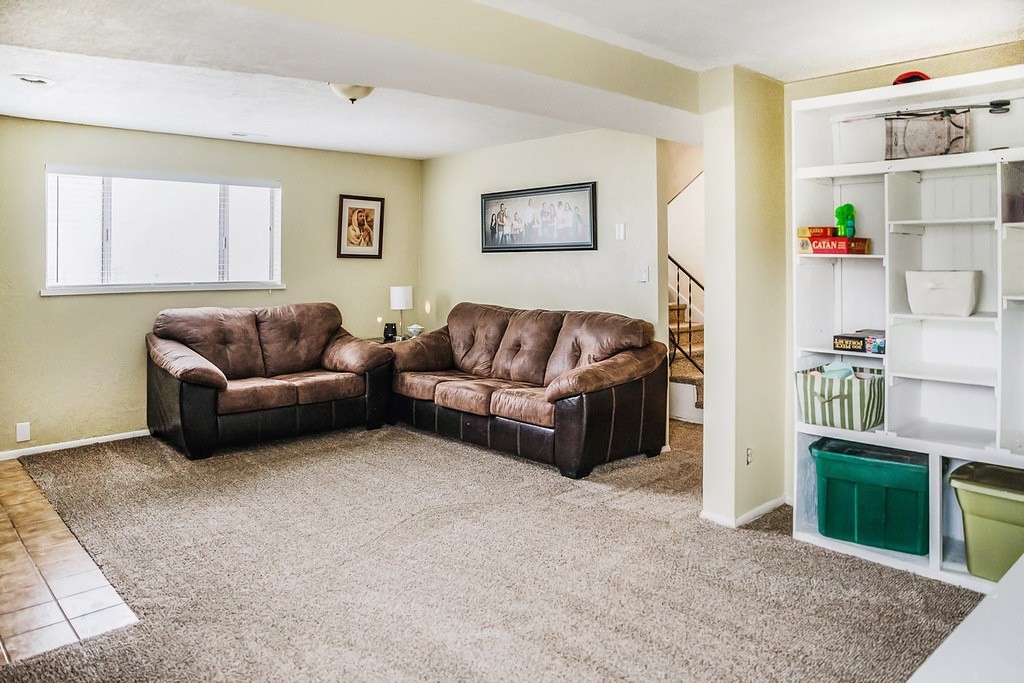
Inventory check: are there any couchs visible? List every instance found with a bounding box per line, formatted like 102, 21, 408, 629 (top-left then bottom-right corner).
145, 301, 394, 459
381, 301, 668, 479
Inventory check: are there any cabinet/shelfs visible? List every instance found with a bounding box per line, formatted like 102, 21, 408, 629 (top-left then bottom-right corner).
790, 64, 1024, 595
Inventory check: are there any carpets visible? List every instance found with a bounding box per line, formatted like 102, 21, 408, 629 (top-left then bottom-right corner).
1, 421, 984, 683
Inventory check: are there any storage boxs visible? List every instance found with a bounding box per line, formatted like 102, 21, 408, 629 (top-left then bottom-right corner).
796, 226, 870, 255
797, 364, 885, 431
949, 463, 1024, 584
806, 437, 928, 556
904, 270, 984, 317
834, 330, 884, 355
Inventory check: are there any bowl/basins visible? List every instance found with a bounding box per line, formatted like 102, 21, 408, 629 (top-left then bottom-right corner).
407, 323, 424, 339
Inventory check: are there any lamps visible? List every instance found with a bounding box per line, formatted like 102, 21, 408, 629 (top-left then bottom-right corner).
390, 286, 414, 339
327, 82, 375, 105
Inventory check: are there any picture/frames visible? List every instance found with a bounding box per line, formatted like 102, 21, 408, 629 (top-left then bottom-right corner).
480, 181, 597, 253
338, 192, 385, 260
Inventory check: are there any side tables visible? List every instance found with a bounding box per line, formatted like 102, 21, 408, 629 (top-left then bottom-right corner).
368, 335, 405, 348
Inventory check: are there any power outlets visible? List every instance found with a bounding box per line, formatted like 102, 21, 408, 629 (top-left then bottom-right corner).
746, 448, 754, 465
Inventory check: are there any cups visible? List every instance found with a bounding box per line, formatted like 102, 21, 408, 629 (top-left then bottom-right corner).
384, 323, 397, 339
